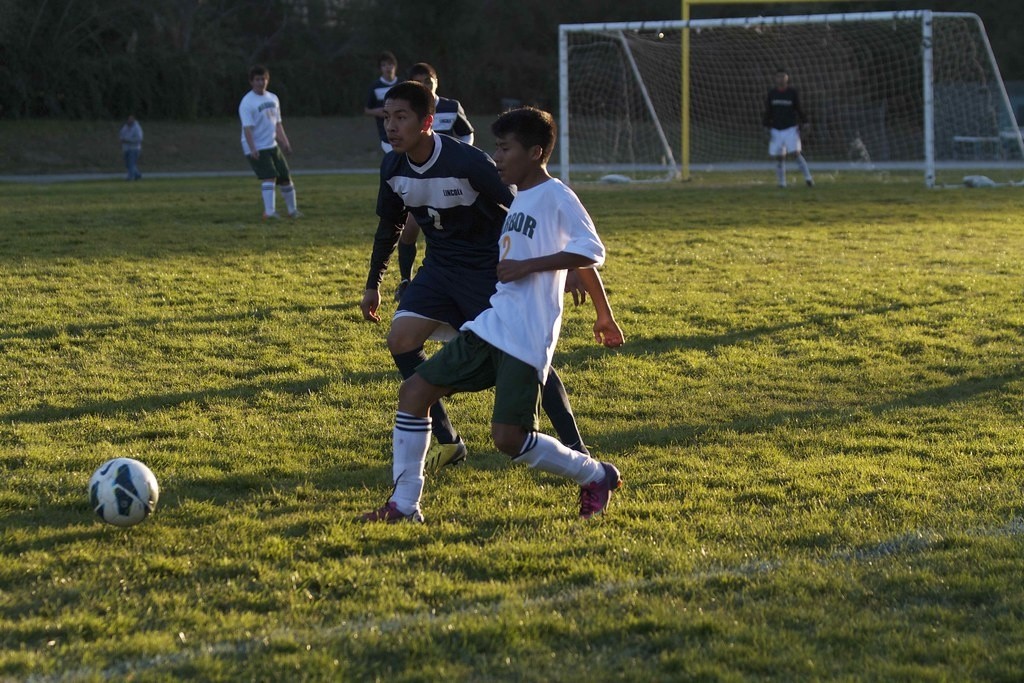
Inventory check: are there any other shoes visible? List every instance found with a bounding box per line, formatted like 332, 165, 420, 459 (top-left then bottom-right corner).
262, 211, 283, 225
807, 176, 814, 186
287, 210, 303, 224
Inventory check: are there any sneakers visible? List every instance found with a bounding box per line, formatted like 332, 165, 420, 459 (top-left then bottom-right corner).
352, 469, 425, 527
424, 434, 468, 475
394, 276, 410, 303
579, 461, 622, 524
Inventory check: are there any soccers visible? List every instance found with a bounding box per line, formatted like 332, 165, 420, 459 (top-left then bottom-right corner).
89, 456, 160, 528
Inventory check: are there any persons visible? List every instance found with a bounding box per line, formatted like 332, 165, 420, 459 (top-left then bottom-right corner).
360, 81, 591, 473
364, 52, 403, 153
238, 65, 303, 219
119, 112, 144, 182
763, 72, 814, 188
394, 63, 474, 301
351, 107, 623, 527
